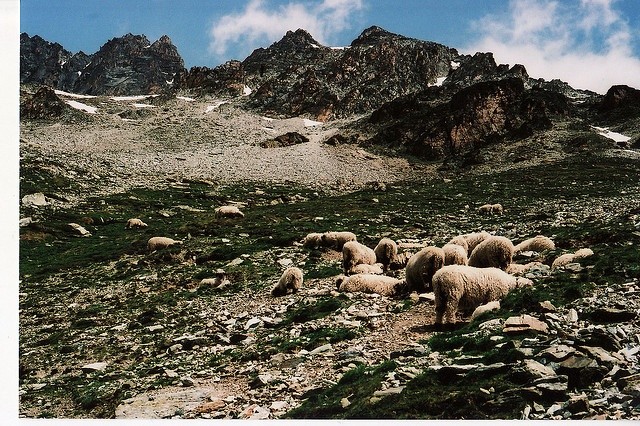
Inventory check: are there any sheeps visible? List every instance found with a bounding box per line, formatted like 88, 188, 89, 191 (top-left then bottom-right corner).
406, 245, 445, 290
493, 203, 503, 215
339, 272, 406, 295
468, 235, 514, 273
304, 232, 322, 244
322, 230, 357, 245
148, 236, 183, 253
432, 263, 534, 324
515, 234, 555, 252
449, 229, 492, 255
219, 205, 245, 217
374, 237, 397, 270
349, 262, 384, 273
343, 240, 377, 277
442, 243, 468, 265
271, 266, 303, 297
214, 207, 220, 218
124, 217, 148, 229
479, 203, 493, 215
